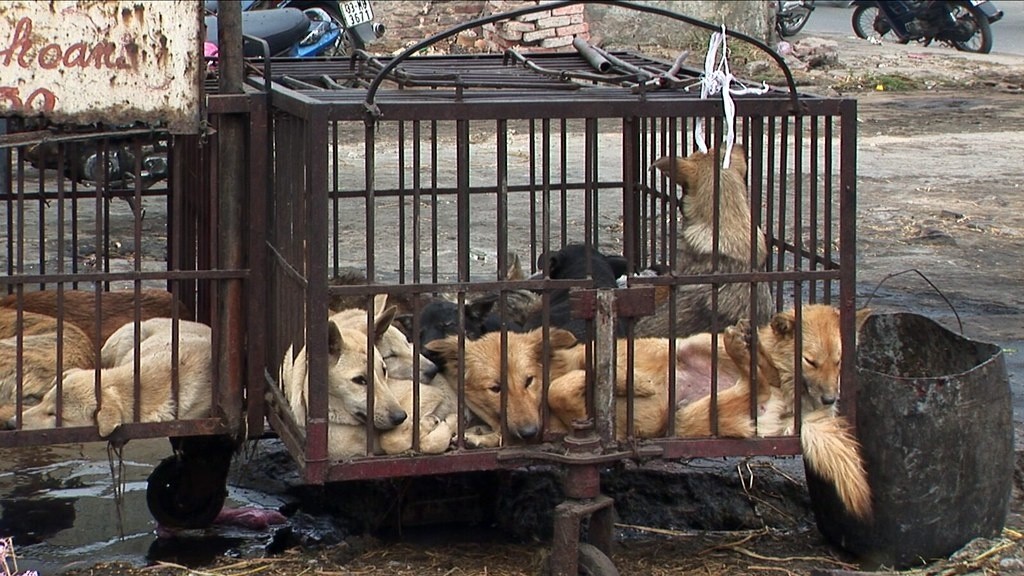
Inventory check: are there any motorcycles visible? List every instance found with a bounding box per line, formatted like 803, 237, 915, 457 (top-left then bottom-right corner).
848, 0, 1004, 55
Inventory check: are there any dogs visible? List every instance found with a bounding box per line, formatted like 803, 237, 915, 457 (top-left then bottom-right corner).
425, 318, 874, 522
6, 317, 213, 438
0, 306, 97, 430
754, 306, 878, 411
633, 142, 773, 338
277, 243, 671, 458
0, 290, 186, 350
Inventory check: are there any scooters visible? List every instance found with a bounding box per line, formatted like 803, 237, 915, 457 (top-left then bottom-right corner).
775, 0, 818, 42
203, 0, 384, 90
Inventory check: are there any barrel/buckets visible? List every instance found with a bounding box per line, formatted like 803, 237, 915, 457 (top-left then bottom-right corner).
803, 269, 1013, 574
